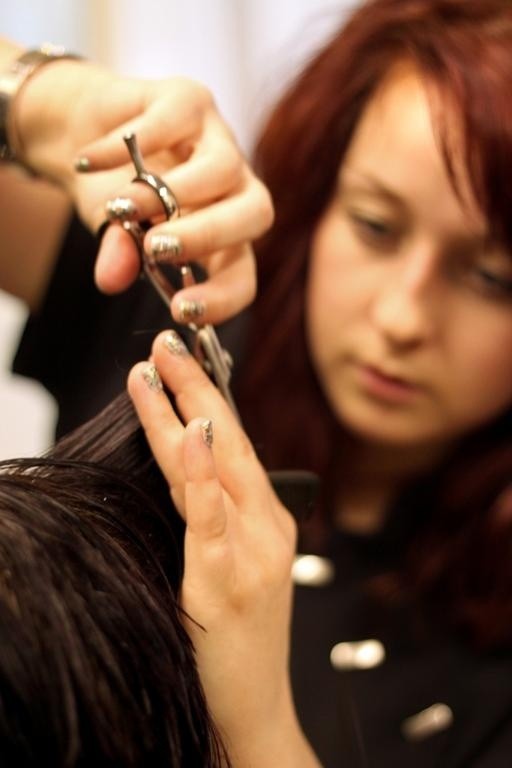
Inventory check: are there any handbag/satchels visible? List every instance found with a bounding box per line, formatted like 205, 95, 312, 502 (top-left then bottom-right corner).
0, 42, 83, 182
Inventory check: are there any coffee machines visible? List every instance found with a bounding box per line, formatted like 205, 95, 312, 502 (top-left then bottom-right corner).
105, 132, 244, 428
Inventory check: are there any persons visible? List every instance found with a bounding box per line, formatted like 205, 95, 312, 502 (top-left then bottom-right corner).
0, 448, 224, 767
0, 0, 512, 767
